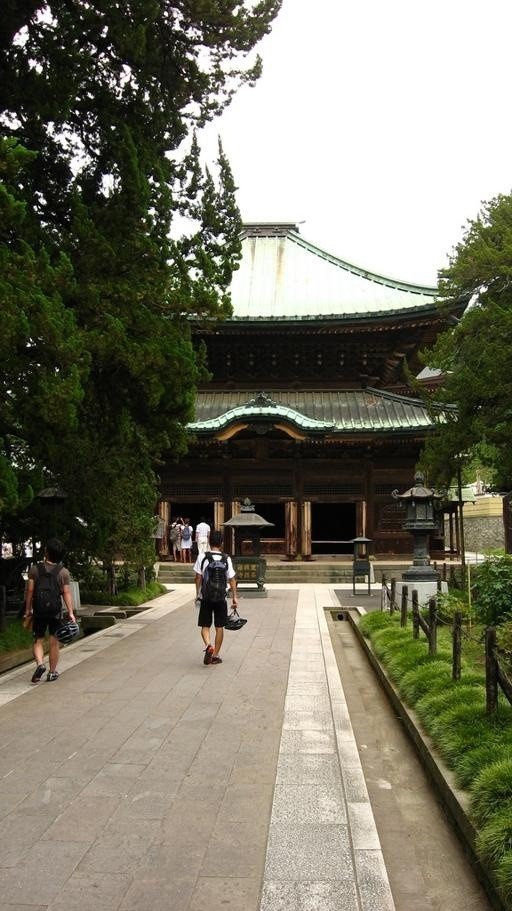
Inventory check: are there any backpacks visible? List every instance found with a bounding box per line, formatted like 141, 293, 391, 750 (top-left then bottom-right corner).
199, 551, 231, 604
169, 523, 181, 543
181, 524, 192, 540
31, 560, 65, 616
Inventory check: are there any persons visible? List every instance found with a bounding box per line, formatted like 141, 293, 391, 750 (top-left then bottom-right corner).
169, 516, 211, 563
23, 539, 76, 680
193, 530, 238, 664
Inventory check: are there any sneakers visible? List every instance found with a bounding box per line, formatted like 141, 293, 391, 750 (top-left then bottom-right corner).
46, 670, 59, 682
203, 645, 214, 665
31, 664, 47, 682
211, 656, 223, 664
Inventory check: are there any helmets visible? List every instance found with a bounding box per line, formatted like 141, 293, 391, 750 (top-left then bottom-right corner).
224, 616, 247, 631
55, 617, 79, 644
